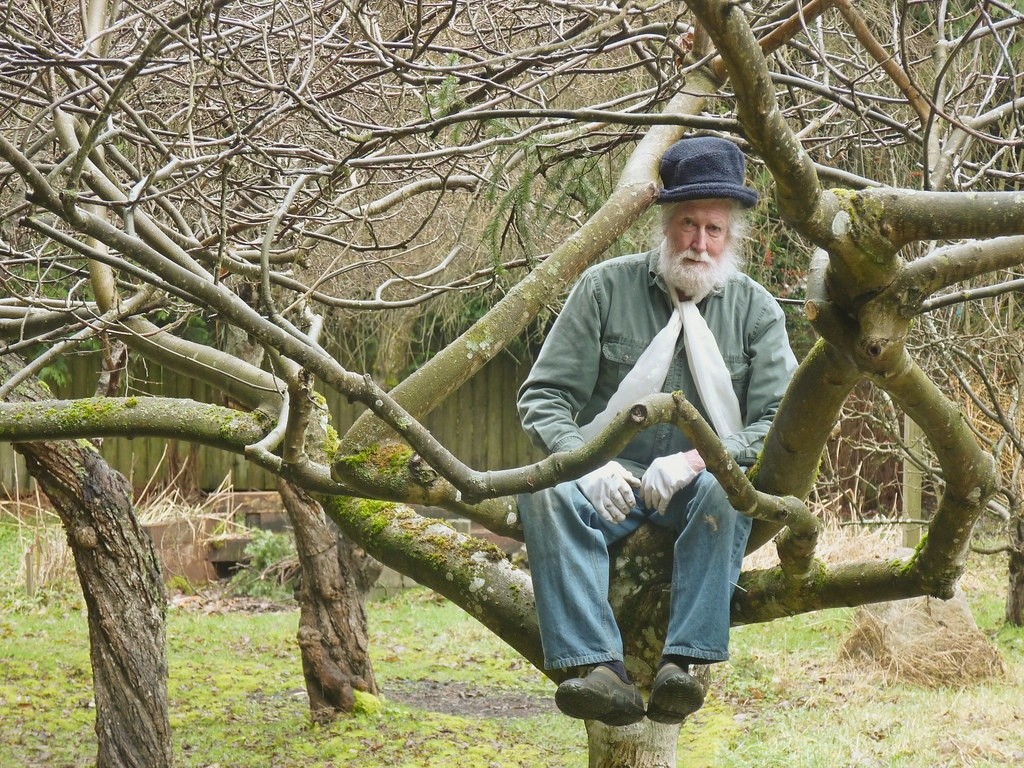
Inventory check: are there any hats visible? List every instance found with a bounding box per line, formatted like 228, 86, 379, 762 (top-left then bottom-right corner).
652, 137, 758, 209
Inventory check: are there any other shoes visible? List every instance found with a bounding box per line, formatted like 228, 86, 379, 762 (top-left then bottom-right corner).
555, 666, 646, 727
646, 663, 704, 724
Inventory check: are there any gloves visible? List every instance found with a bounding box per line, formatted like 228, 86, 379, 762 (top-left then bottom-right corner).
577, 460, 642, 525
640, 451, 697, 516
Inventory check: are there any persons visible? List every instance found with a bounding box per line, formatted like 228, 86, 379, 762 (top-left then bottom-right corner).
517, 138, 800, 726
